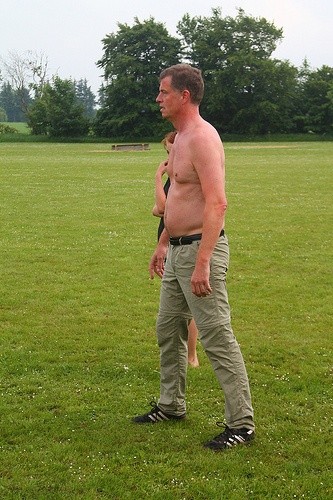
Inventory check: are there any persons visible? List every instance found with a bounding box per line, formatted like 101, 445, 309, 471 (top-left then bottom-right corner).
132, 64, 255, 452
154, 131, 200, 368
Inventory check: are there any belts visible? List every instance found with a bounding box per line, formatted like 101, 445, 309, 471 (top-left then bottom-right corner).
170, 230, 225, 246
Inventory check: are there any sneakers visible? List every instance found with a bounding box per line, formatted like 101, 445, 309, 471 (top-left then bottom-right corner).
203, 421, 256, 450
132, 402, 186, 423
